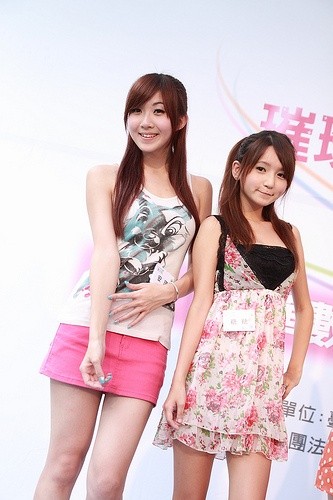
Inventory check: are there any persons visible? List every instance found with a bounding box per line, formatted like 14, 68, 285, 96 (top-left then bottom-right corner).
34, 73, 212, 500
154, 129, 315, 500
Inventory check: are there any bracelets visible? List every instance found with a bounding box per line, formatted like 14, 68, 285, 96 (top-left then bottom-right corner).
169, 283, 179, 300
